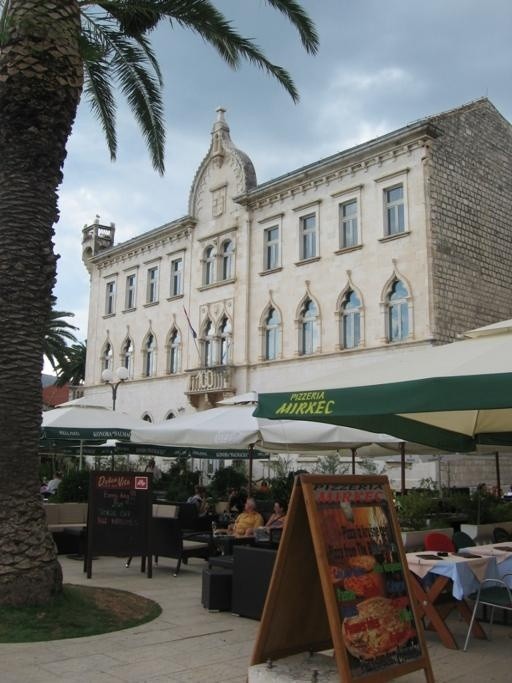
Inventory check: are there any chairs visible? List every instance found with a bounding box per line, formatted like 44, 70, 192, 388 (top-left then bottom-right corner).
450, 531, 478, 551
199, 516, 283, 621
491, 526, 510, 543
421, 531, 458, 555
43, 501, 211, 581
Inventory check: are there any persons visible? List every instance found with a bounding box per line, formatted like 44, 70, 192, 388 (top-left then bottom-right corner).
41, 460, 289, 556
472, 483, 492, 501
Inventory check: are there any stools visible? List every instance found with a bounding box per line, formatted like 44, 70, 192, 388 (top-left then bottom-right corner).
460, 572, 512, 653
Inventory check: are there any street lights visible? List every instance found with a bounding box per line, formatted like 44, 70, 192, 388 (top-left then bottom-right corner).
100, 364, 129, 471
